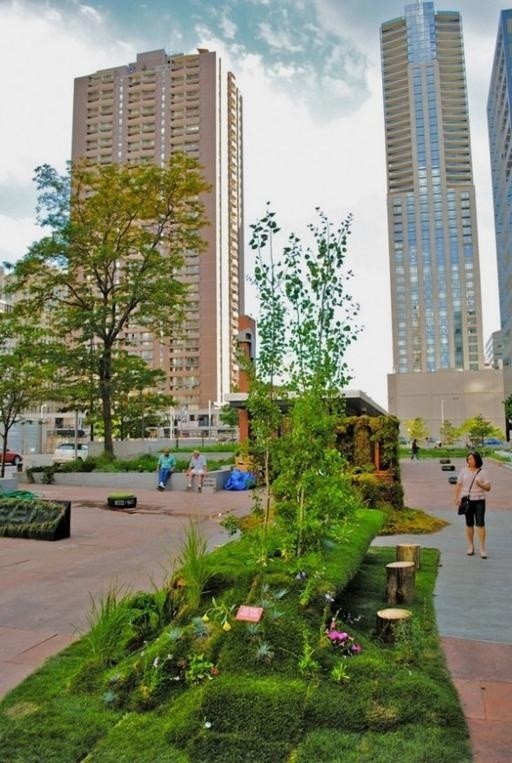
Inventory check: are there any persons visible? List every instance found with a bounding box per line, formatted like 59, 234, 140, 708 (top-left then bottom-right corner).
453, 449, 492, 559
185, 449, 208, 493
408, 438, 422, 463
154, 446, 177, 490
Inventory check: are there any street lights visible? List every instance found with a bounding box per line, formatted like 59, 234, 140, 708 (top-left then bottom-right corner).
440, 396, 461, 448
207, 400, 218, 440
41, 403, 48, 419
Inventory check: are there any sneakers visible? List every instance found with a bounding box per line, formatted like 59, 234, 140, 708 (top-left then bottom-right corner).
155, 482, 202, 492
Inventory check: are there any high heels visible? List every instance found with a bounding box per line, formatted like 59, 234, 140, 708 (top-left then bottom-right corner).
467, 546, 488, 559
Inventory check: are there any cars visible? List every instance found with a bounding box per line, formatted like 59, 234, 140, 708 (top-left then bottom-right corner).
0, 445, 24, 468
480, 438, 502, 445
50, 442, 90, 464
398, 435, 437, 446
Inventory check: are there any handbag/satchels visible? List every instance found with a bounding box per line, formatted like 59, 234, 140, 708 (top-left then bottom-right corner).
458, 496, 471, 515
